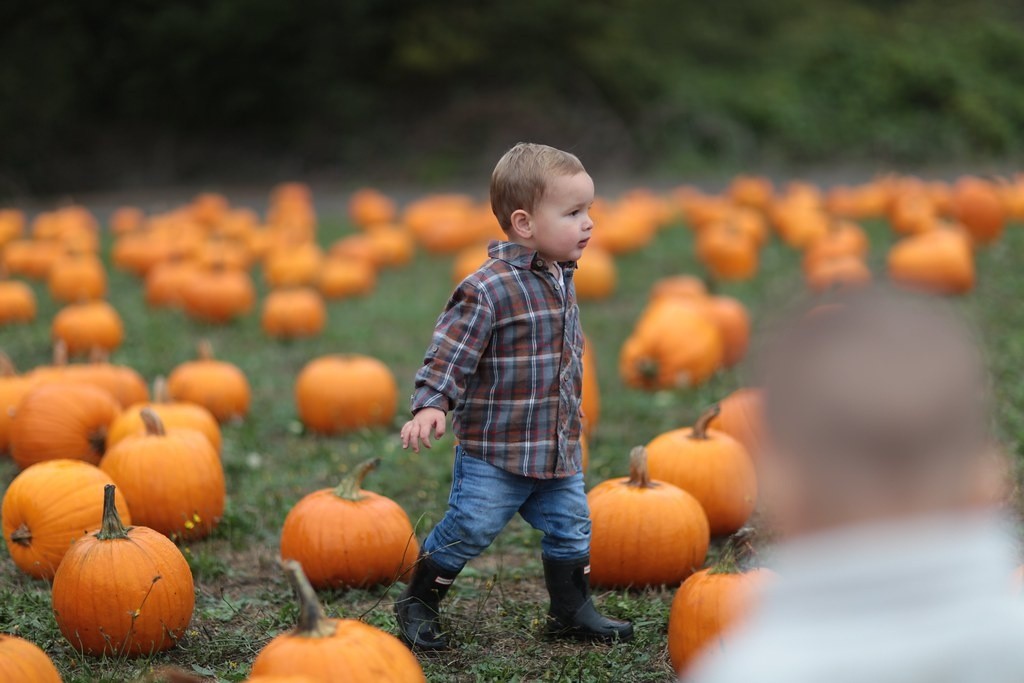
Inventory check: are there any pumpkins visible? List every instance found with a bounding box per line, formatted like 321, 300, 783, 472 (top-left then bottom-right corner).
0, 169, 1024, 683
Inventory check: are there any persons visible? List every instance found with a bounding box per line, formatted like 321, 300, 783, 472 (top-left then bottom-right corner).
661, 278, 1024, 683
396, 142, 638, 651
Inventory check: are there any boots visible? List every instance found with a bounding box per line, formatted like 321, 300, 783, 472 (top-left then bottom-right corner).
541, 552, 636, 643
393, 537, 466, 651
